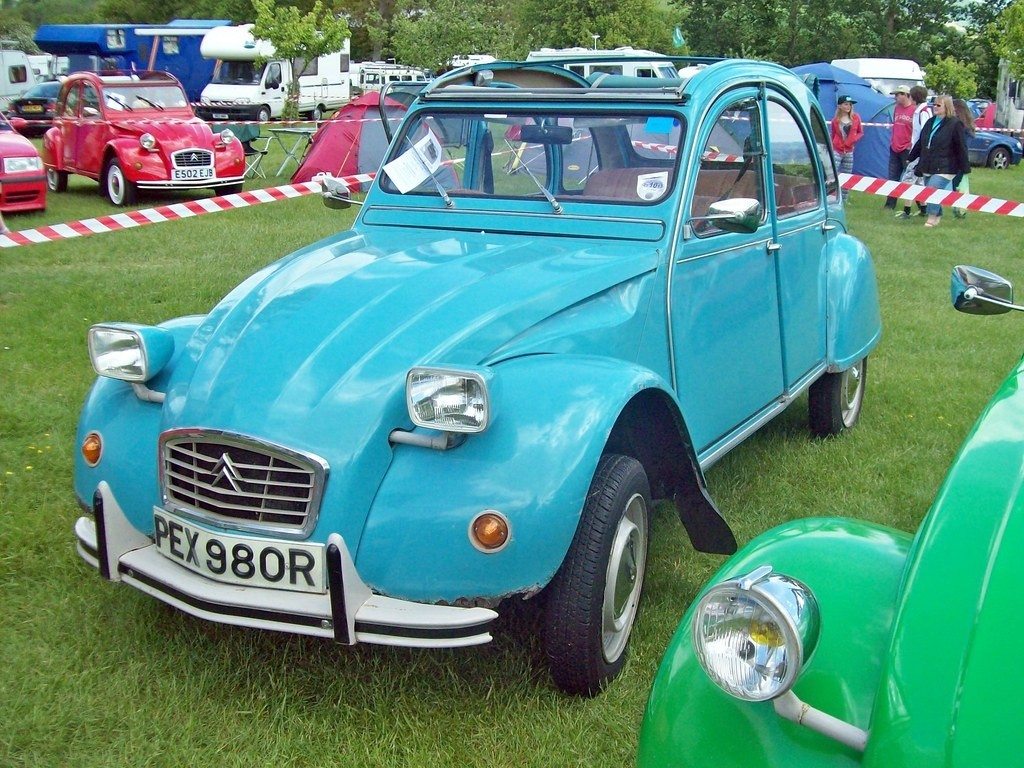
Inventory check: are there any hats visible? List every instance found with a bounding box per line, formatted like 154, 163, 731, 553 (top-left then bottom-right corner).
889, 85, 910, 94
838, 95, 858, 104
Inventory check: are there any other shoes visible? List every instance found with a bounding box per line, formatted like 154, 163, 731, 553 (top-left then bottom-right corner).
925, 215, 940, 227
893, 211, 912, 219
913, 211, 927, 217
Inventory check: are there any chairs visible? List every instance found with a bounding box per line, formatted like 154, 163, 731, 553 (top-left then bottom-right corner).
213, 123, 269, 180
240, 65, 254, 82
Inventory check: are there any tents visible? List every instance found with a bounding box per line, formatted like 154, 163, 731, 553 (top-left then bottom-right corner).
289, 92, 461, 192
387, 85, 463, 147
502, 118, 744, 181
789, 63, 896, 183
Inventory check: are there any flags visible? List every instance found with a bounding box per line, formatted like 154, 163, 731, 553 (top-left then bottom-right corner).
672, 25, 685, 48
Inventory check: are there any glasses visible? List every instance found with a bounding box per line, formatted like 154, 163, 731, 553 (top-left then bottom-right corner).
933, 103, 941, 107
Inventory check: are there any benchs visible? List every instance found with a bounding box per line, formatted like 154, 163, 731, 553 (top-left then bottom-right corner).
581, 167, 809, 228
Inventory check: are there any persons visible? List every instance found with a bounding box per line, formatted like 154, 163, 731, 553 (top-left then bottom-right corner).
895, 86, 933, 219
889, 85, 916, 209
905, 95, 977, 227
829, 95, 864, 202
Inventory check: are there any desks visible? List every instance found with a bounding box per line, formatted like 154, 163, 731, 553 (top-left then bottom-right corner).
268, 128, 321, 178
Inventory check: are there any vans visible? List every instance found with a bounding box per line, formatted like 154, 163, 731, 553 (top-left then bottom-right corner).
829, 58, 929, 100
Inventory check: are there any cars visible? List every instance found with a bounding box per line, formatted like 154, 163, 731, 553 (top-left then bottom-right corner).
43, 66, 247, 207
0, 112, 47, 216
71, 55, 883, 702
0, 23, 1024, 155
632, 261, 1023, 768
921, 94, 1024, 173
